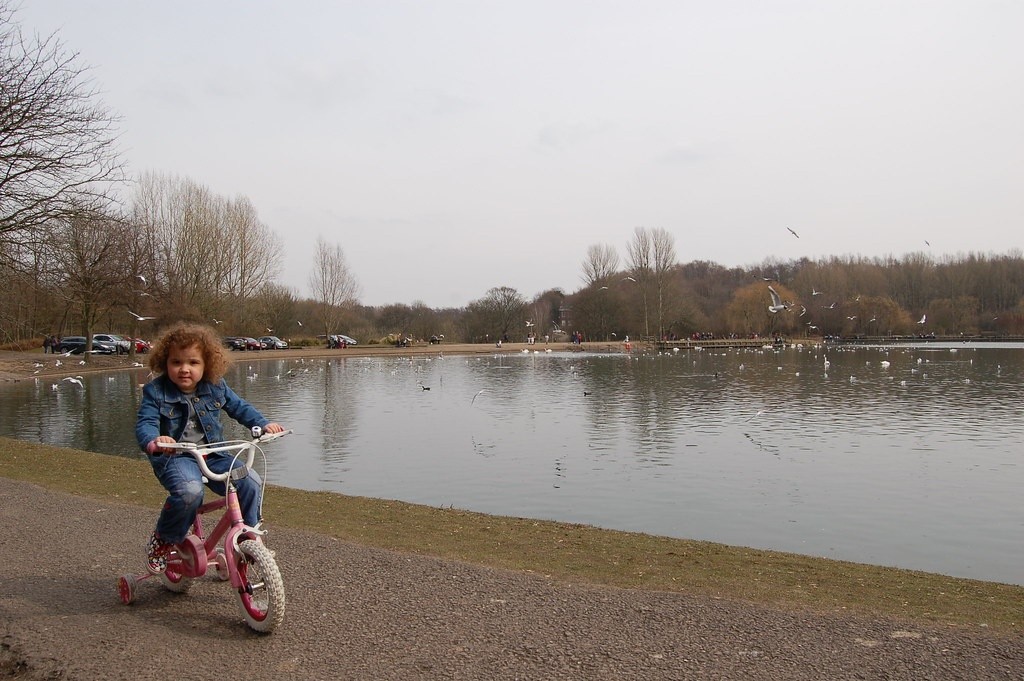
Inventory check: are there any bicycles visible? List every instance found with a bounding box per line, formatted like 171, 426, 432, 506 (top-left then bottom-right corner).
118, 428, 294, 634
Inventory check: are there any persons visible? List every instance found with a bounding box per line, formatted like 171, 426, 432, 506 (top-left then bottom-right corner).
572, 330, 582, 345
692, 331, 713, 340
329, 334, 348, 349
135, 321, 285, 575
431, 334, 437, 345
42, 335, 58, 353
722, 332, 738, 341
527, 331, 549, 345
396, 333, 408, 348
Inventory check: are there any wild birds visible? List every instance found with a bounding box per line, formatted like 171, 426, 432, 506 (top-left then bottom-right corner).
33, 347, 86, 390
462, 331, 1024, 425
211, 287, 304, 333
525, 223, 930, 331
357, 352, 445, 391
124, 273, 159, 322
246, 356, 332, 381
106, 361, 154, 387
470, 388, 486, 406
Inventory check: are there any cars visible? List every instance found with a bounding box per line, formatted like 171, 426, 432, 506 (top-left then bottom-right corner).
239, 336, 268, 350
122, 335, 152, 354
223, 338, 246, 351
257, 336, 289, 350
316, 333, 357, 344
54, 336, 113, 355
92, 334, 131, 354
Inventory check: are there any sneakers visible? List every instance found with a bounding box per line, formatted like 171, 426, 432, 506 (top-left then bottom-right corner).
144, 531, 174, 575
